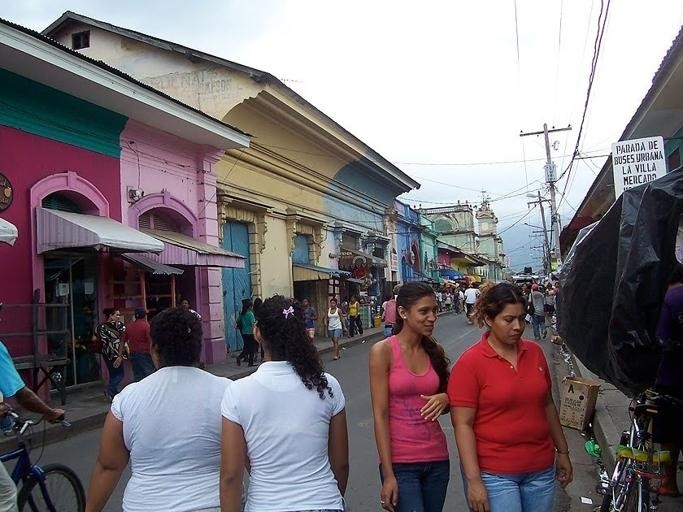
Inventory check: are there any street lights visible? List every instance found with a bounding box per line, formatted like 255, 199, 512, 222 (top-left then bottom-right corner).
527, 191, 552, 216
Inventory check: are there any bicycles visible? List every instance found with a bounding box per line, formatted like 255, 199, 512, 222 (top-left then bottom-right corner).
0, 406, 85, 512
600, 387, 675, 511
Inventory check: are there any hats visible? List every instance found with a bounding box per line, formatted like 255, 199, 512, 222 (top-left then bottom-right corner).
134, 309, 149, 317
532, 283, 539, 290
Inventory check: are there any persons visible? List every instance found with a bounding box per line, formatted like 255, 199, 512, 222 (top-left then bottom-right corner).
515, 281, 561, 341
381, 281, 482, 339
89, 298, 203, 404
369, 280, 450, 512
234, 291, 363, 368
447, 281, 574, 512
1, 341, 65, 512
83, 308, 233, 512
651, 262, 683, 498
218, 295, 348, 512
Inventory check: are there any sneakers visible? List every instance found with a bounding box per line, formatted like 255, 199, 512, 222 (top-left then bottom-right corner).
2, 422, 16, 435
535, 337, 541, 341
237, 351, 258, 366
542, 330, 548, 339
102, 387, 112, 402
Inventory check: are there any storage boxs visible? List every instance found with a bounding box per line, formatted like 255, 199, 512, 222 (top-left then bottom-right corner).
561, 375, 602, 429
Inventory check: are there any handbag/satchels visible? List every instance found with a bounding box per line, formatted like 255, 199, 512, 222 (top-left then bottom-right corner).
529, 304, 535, 316
91, 340, 102, 354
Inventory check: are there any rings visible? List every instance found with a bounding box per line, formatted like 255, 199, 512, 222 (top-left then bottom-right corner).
381, 501, 384, 504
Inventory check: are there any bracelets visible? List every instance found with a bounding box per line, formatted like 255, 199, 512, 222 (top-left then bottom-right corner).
555, 448, 570, 454
